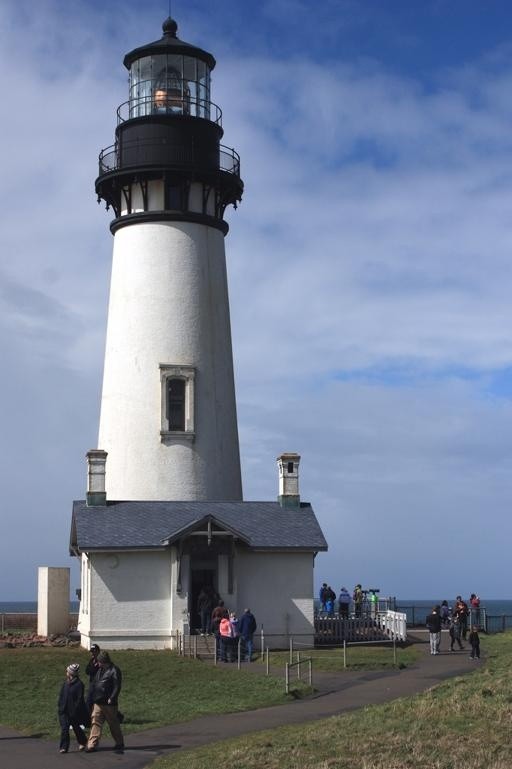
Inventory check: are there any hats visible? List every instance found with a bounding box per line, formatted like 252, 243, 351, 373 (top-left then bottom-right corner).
67, 664, 80, 676
90, 644, 99, 652
97, 650, 110, 663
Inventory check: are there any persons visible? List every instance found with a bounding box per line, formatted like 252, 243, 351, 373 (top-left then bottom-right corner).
353, 584, 363, 617
426, 594, 481, 660
86, 650, 125, 754
195, 580, 257, 664
86, 644, 124, 727
338, 586, 351, 620
319, 583, 336, 618
57, 664, 88, 754
